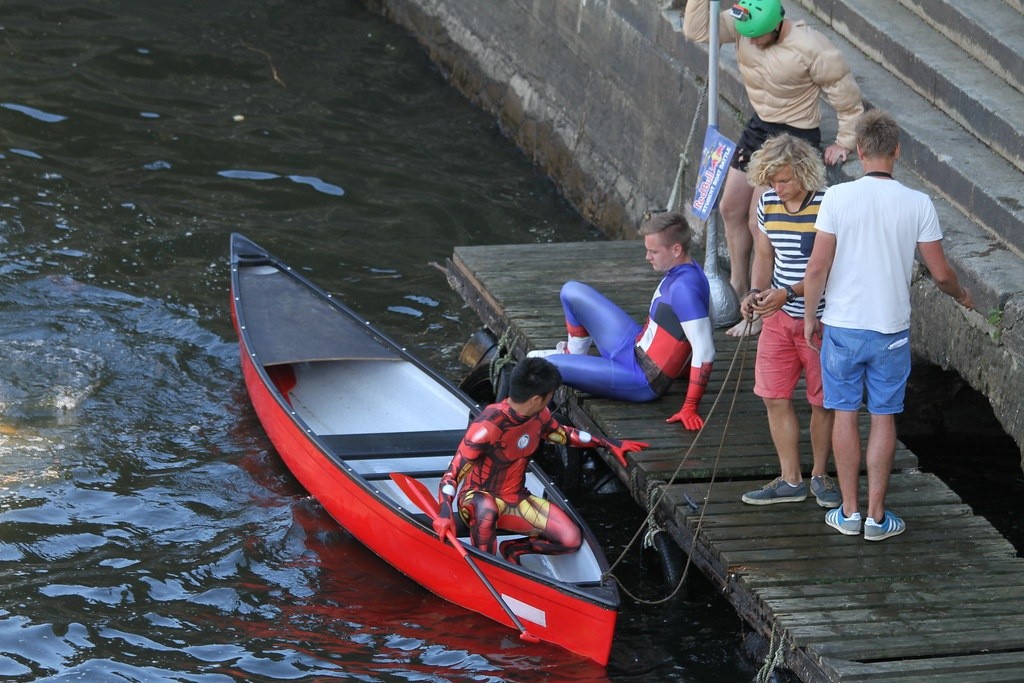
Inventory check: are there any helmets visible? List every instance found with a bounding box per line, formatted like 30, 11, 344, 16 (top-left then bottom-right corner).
735, 0, 785, 38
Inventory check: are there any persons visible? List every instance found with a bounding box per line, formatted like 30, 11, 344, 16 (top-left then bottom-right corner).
741, 133, 843, 508
433, 357, 650, 564
804, 108, 973, 541
527, 211, 716, 431
683, 0, 865, 337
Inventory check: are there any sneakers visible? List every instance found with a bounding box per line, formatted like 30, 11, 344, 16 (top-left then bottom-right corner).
824, 504, 861, 535
864, 509, 906, 541
742, 475, 806, 504
810, 475, 841, 508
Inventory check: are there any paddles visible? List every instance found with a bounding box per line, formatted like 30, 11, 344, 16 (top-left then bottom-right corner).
389, 470, 541, 645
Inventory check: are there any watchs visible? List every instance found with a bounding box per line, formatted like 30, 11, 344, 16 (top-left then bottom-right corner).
786, 286, 796, 302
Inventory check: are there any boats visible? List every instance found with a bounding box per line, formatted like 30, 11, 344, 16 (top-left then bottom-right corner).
229, 232, 618, 665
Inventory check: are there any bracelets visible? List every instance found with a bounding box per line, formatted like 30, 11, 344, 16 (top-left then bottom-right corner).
960, 288, 966, 302
749, 289, 764, 301
